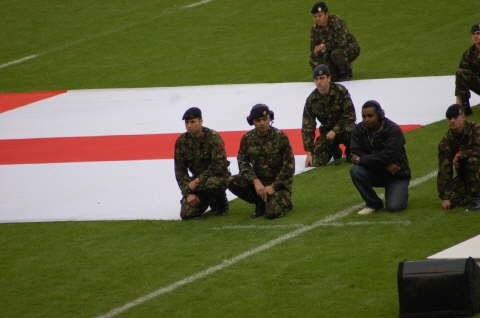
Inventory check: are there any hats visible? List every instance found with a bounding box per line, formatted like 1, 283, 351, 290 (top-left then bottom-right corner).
471, 23, 480, 33
311, 2, 328, 14
182, 107, 202, 120
313, 64, 330, 78
446, 104, 463, 118
251, 103, 270, 120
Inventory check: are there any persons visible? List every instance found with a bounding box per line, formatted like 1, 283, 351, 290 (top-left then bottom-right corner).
437, 104, 480, 211
309, 2, 360, 81
455, 25, 480, 117
303, 64, 356, 167
174, 107, 230, 219
347, 101, 411, 216
229, 104, 295, 219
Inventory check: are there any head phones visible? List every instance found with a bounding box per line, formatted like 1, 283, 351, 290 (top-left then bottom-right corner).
369, 100, 384, 118
247, 104, 275, 125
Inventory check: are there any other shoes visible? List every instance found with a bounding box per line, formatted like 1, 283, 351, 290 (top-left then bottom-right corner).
334, 150, 343, 165
255, 205, 264, 217
357, 207, 375, 216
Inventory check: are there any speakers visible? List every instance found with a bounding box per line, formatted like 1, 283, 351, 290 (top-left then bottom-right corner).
398, 256, 480, 318
